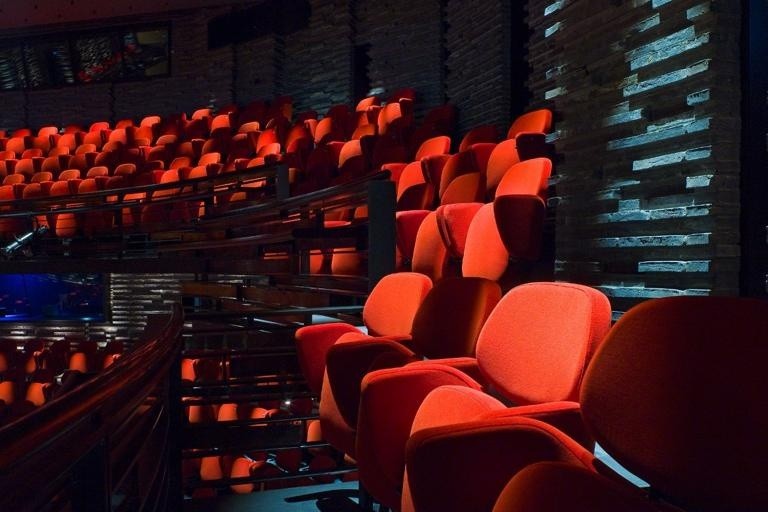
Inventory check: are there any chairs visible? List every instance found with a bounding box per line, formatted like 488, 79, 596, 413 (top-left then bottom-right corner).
0, 93, 767, 512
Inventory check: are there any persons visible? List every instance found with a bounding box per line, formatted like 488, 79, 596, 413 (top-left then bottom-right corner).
116, 32, 154, 78
